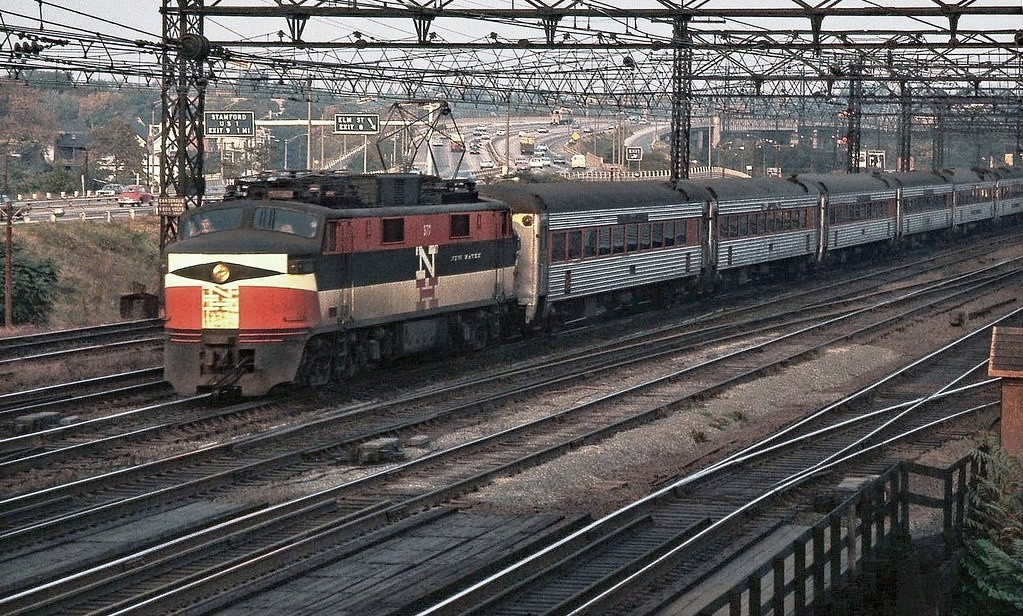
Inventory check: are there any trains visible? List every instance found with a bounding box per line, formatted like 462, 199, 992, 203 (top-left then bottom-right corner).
162, 165, 1023, 397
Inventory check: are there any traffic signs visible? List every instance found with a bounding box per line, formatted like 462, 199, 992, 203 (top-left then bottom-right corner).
626, 147, 642, 161
334, 114, 379, 135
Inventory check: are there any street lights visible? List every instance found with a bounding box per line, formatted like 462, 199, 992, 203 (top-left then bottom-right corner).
284, 134, 308, 170
323, 136, 342, 169
746, 135, 766, 177
717, 142, 733, 178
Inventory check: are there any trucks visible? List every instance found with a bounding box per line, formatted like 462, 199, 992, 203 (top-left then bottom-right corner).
519, 136, 534, 155
450, 134, 464, 151
537, 126, 548, 132
571, 155, 586, 169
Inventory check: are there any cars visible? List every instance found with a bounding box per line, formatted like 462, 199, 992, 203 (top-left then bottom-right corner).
432, 134, 446, 146
514, 143, 565, 172
455, 170, 476, 180
570, 122, 579, 128
479, 159, 493, 169
629, 116, 651, 125
470, 124, 506, 154
96, 184, 124, 201
117, 186, 154, 207
456, 130, 465, 134
583, 125, 593, 133
